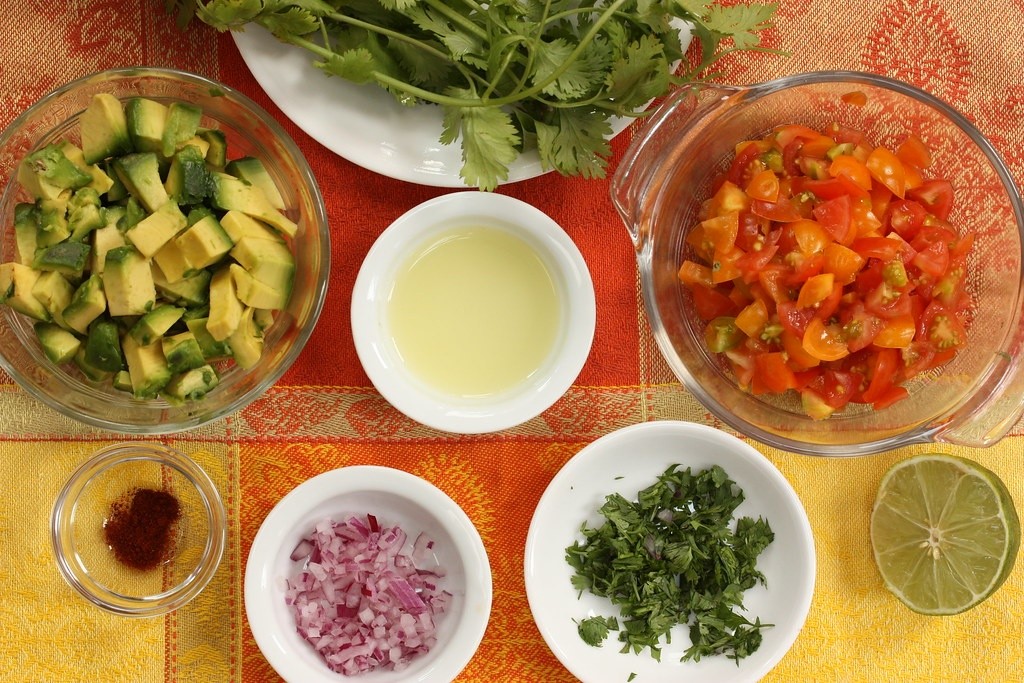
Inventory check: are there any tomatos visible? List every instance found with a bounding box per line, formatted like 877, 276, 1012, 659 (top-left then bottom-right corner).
676, 123, 975, 423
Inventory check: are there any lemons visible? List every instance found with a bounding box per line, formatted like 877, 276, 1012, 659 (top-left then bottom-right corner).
871, 452, 1019, 615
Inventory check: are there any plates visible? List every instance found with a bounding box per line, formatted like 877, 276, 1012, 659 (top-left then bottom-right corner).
244, 465, 492, 683
524, 421, 816, 683
230, 13, 696, 187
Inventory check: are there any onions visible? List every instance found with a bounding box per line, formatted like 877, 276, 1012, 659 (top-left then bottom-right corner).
285, 513, 451, 677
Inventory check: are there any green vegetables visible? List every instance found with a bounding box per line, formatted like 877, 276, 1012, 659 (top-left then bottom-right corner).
152, 0, 795, 194
565, 462, 776, 682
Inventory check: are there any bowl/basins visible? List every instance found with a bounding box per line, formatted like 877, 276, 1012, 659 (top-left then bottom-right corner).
351, 190, 596, 434
49, 441, 226, 617
0, 66, 329, 433
609, 70, 1024, 460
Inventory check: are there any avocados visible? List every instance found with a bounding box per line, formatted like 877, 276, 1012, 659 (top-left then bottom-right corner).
0, 93, 297, 407
